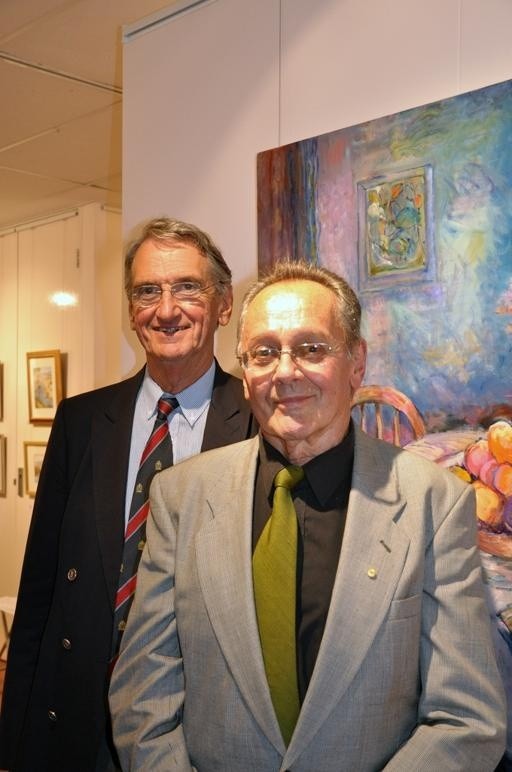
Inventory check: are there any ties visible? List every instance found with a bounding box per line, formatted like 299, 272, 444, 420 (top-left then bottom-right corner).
252, 469, 302, 750
110, 397, 178, 675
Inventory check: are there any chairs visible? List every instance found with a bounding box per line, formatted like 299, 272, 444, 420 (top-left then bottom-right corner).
342, 386, 425, 450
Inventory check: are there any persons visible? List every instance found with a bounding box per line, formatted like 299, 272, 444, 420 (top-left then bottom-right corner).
107, 257, 509, 772
0, 217, 259, 772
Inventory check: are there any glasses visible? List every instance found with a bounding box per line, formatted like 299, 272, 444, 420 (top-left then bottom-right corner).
131, 281, 204, 307
242, 342, 340, 366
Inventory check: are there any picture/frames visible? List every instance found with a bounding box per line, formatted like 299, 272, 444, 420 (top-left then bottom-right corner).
24, 346, 64, 500
355, 164, 434, 292
